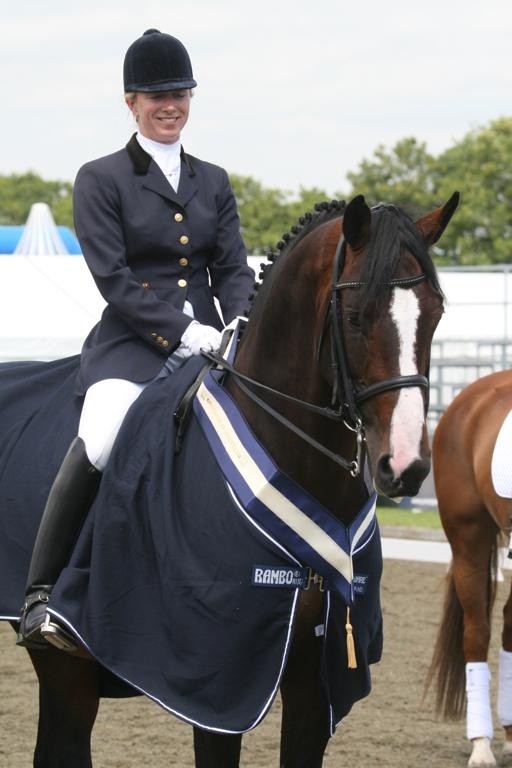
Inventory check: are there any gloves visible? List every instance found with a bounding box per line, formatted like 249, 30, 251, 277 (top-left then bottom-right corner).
181, 318, 223, 356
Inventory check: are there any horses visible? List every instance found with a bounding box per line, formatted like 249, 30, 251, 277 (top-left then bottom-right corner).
2, 188, 461, 768
421, 367, 512, 768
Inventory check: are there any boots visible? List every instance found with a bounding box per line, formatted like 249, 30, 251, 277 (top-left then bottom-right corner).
16, 434, 103, 651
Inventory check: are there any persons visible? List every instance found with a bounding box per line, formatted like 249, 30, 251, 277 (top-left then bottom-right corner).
15, 27, 263, 653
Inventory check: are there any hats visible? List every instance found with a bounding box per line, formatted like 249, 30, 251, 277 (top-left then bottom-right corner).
122, 27, 198, 93
